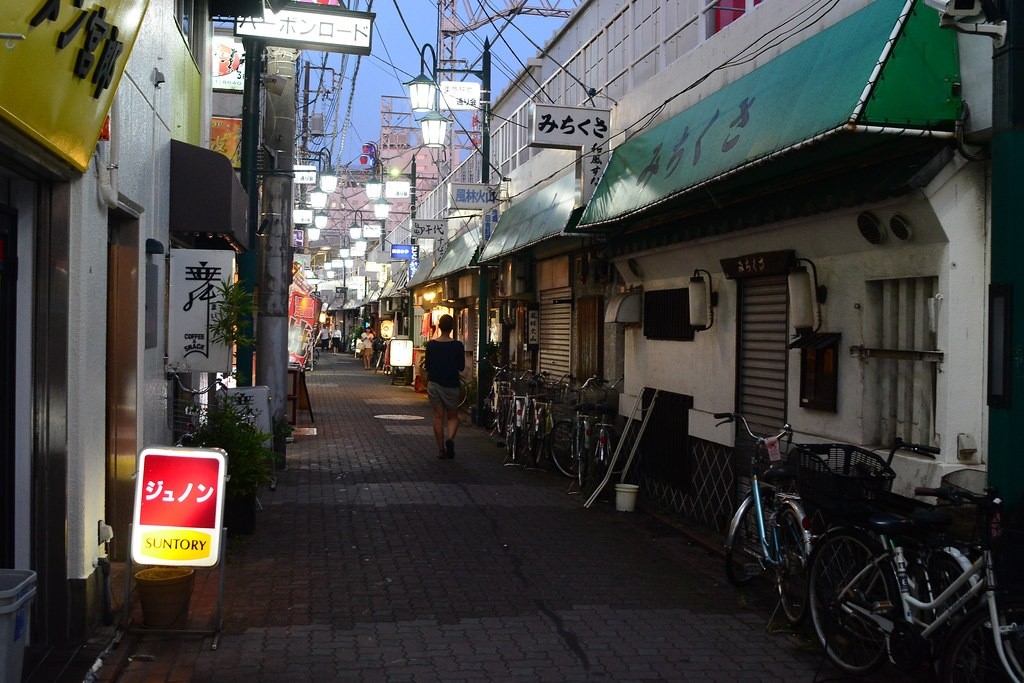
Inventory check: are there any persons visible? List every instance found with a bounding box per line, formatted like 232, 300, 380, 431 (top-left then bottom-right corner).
320, 324, 331, 353
331, 325, 342, 356
361, 327, 374, 370
425, 314, 465, 459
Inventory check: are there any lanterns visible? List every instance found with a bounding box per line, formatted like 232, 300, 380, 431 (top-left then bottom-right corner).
432, 304, 454, 326
319, 311, 327, 323
380, 320, 394, 339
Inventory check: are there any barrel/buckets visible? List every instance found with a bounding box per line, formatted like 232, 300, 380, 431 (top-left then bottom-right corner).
615, 484, 639, 512
134, 566, 195, 630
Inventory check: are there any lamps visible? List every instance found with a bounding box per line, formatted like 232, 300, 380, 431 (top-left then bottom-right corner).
787, 258, 827, 340
688, 268, 718, 332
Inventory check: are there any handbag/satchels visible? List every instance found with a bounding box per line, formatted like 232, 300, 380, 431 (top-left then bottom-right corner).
358, 340, 365, 350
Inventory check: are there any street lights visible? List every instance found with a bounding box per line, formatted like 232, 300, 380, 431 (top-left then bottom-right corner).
401, 46, 487, 403
294, 146, 416, 386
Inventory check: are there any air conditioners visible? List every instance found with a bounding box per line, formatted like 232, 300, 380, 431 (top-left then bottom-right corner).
498, 257, 526, 298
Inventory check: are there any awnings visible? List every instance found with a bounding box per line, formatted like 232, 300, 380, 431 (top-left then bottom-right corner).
357, 270, 410, 307
405, 228, 499, 290
169, 139, 249, 254
477, 191, 613, 264
328, 297, 360, 310
604, 292, 641, 323
575, 0, 962, 229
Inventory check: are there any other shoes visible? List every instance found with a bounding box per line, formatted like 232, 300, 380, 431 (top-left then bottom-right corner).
333, 353, 336, 356
445, 440, 454, 458
365, 366, 371, 370
433, 447, 444, 458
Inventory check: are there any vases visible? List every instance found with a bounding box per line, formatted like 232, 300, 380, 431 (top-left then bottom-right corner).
135, 567, 195, 630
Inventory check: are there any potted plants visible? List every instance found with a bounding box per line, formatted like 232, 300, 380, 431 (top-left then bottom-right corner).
272, 409, 295, 470
187, 395, 285, 535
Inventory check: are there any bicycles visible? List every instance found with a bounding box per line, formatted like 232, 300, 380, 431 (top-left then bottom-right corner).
480, 357, 624, 502
459, 366, 469, 408
162, 370, 228, 450
714, 411, 1023, 683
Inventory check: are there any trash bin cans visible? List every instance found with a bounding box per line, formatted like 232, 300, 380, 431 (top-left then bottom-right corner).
1, 566, 37, 683
